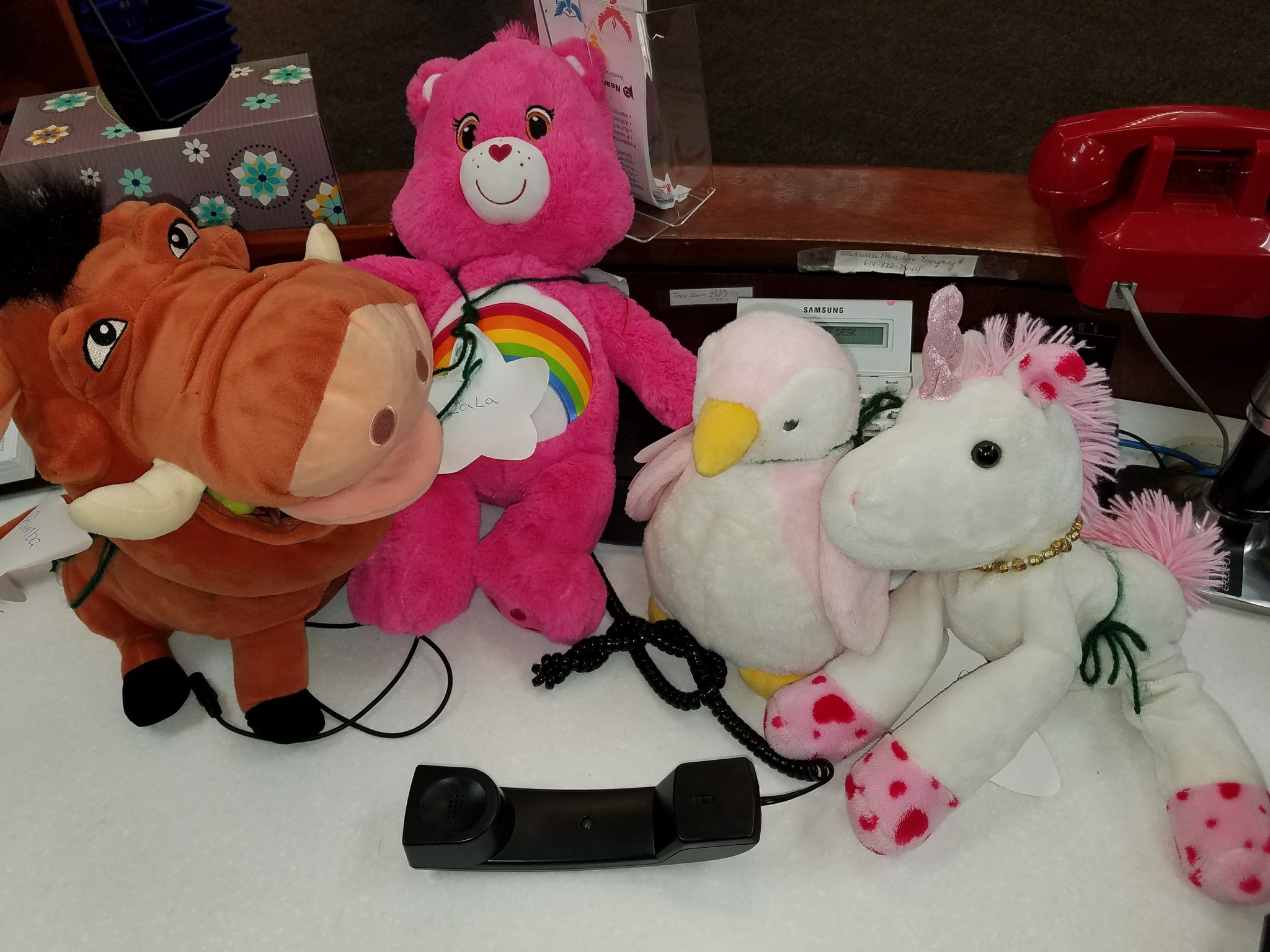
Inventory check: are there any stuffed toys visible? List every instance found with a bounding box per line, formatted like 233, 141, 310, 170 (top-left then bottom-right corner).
1, 199, 441, 744
346, 36, 694, 639
763, 285, 1270, 906
625, 311, 893, 700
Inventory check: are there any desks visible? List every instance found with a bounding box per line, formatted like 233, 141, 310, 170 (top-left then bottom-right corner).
3, 354, 1270, 952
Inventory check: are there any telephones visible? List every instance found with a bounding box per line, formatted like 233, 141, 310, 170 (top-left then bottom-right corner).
402, 757, 762, 872
1026, 104, 1270, 318
